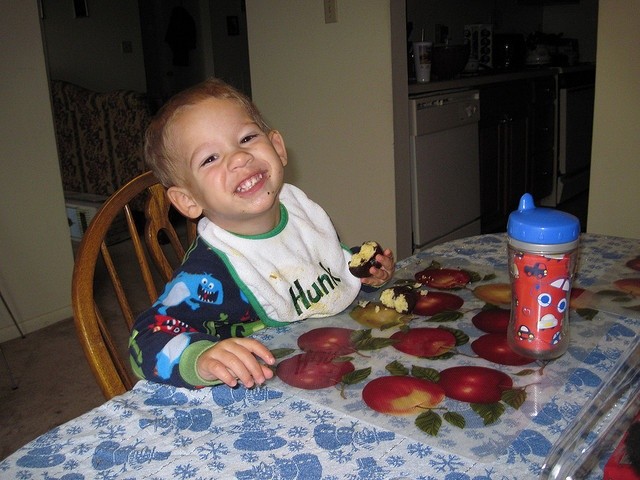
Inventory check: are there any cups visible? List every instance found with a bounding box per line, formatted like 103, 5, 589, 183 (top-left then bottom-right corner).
411, 39, 434, 85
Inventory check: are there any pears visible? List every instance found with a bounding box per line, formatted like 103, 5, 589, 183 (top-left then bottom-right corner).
349, 302, 419, 334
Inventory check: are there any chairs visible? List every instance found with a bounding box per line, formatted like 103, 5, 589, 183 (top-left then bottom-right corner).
66, 172, 212, 401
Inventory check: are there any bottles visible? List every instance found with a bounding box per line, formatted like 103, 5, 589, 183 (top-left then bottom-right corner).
505, 193, 581, 361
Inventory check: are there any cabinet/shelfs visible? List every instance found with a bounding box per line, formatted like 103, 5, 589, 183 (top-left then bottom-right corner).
474, 71, 558, 234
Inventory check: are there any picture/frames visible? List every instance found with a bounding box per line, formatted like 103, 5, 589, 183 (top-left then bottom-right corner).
72, 1, 89, 18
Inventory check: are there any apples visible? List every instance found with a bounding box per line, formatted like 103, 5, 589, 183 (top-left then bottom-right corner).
390, 325, 469, 360
595, 279, 640, 303
626, 258, 640, 271
471, 334, 550, 376
415, 269, 470, 289
569, 288, 592, 309
362, 376, 465, 436
471, 308, 510, 333
276, 353, 371, 399
297, 327, 400, 358
466, 284, 512, 304
436, 366, 541, 426
413, 291, 464, 322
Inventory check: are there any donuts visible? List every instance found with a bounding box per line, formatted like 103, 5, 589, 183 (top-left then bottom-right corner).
379, 286, 414, 315
348, 240, 383, 278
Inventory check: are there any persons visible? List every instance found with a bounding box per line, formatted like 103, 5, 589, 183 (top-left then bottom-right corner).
127, 74, 397, 390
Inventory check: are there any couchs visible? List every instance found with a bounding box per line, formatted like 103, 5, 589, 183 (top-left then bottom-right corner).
49, 80, 193, 244
